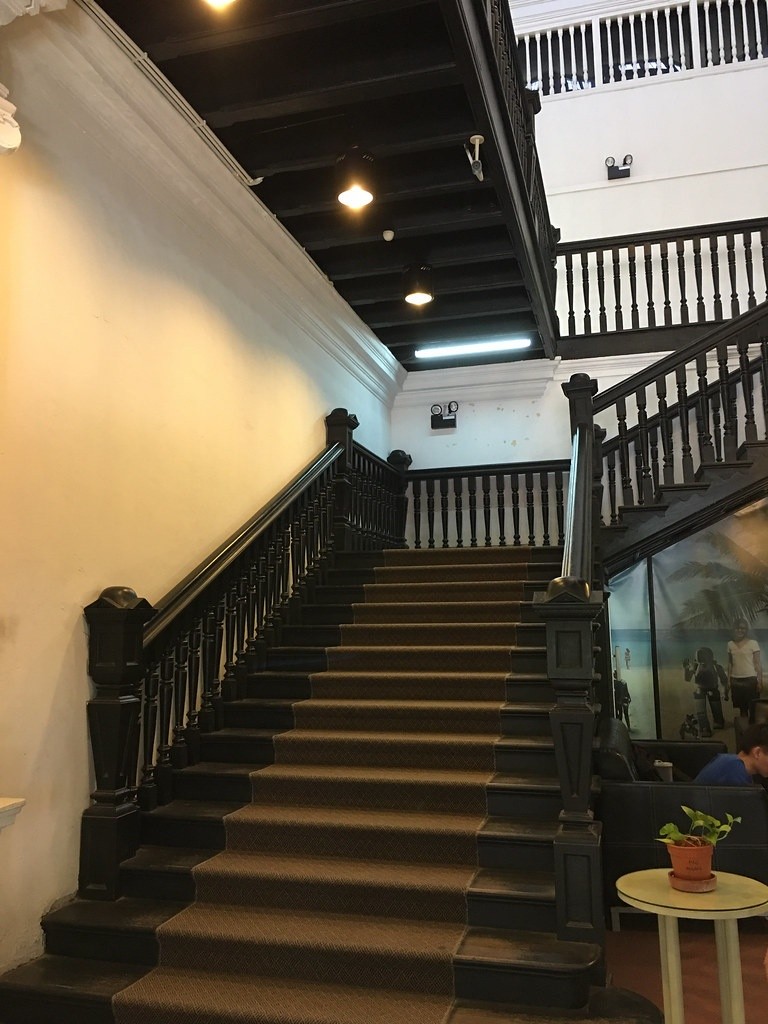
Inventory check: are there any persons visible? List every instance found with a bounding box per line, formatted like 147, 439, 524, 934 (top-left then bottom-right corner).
634, 724, 768, 788
614, 617, 764, 736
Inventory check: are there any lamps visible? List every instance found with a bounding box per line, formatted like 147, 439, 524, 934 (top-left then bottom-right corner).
429, 399, 458, 430
336, 149, 376, 203
396, 264, 435, 304
605, 154, 633, 180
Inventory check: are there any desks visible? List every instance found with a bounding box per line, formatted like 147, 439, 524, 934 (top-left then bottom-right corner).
613, 868, 768, 1024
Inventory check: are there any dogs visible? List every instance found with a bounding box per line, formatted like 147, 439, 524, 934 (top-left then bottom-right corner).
680, 713, 699, 740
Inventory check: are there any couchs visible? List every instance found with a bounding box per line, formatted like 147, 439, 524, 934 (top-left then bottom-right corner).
602, 718, 768, 930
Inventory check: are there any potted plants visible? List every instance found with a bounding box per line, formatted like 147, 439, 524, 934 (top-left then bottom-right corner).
654, 803, 745, 894
